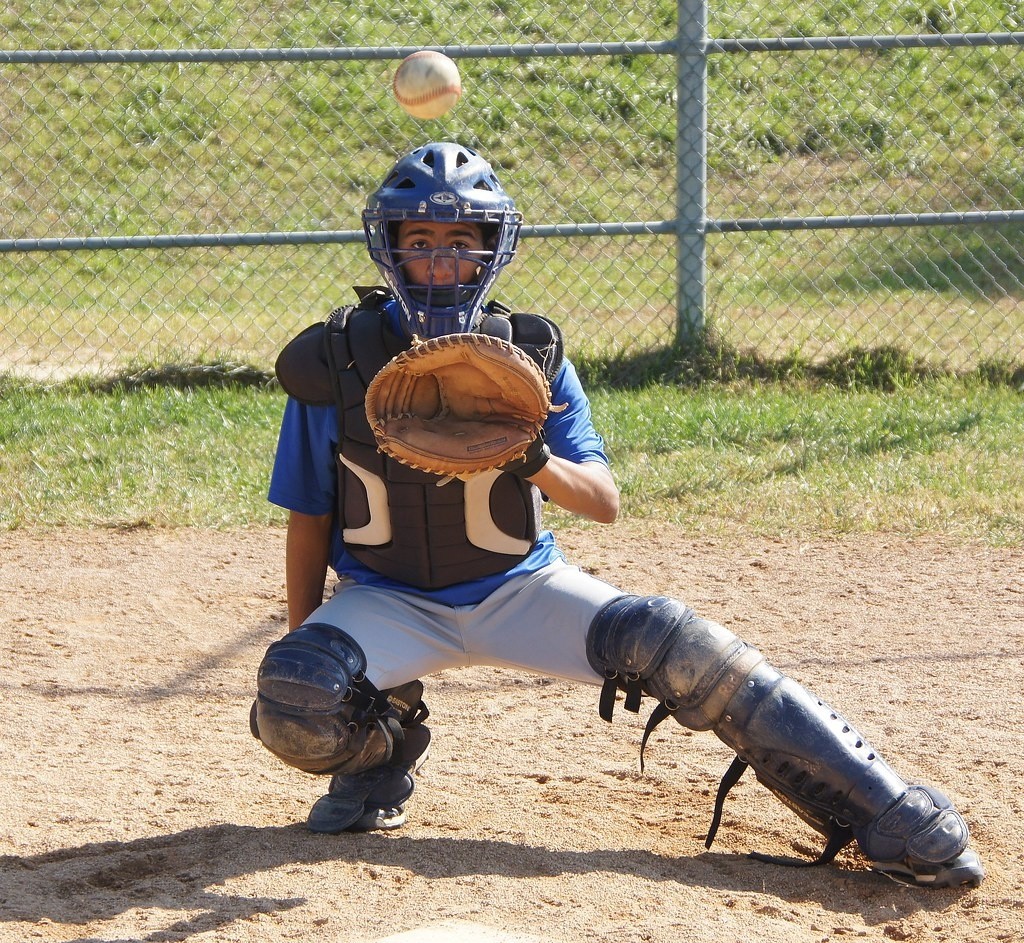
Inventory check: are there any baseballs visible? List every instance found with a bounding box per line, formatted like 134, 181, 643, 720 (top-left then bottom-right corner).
393, 50, 462, 120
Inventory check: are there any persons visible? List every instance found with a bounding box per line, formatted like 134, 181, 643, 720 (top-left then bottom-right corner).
249, 142, 984, 888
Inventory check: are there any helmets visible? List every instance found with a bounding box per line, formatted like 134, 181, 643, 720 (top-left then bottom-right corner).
361, 145, 522, 337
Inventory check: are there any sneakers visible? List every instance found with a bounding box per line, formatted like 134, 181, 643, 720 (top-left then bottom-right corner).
352, 735, 432, 828
868, 865, 971, 892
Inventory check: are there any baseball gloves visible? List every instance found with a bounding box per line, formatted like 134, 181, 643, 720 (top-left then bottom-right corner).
364, 332, 569, 487
